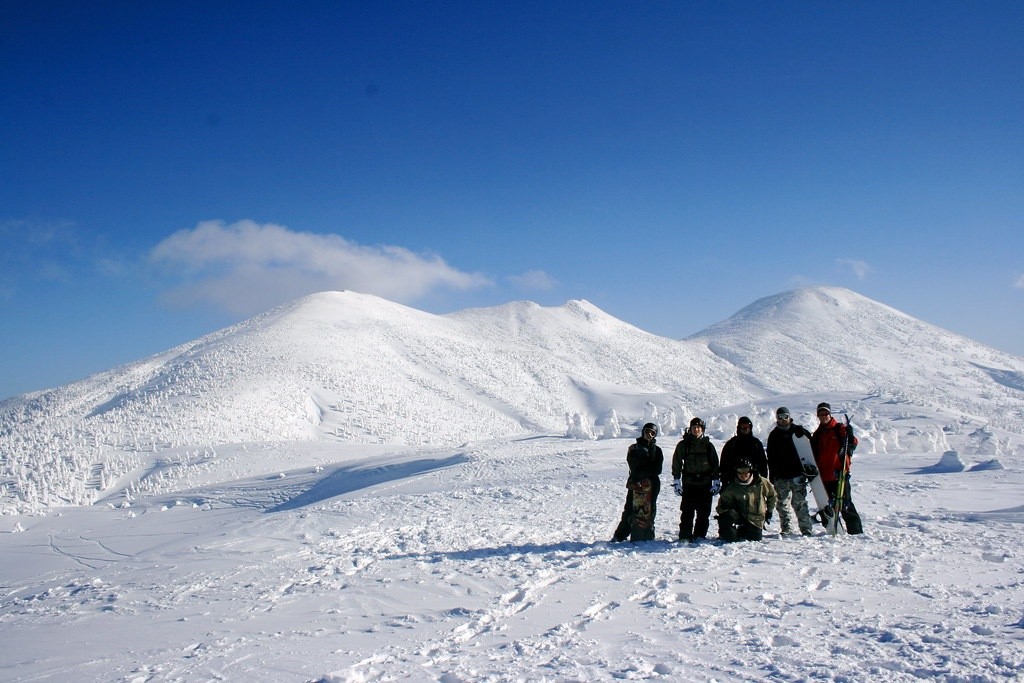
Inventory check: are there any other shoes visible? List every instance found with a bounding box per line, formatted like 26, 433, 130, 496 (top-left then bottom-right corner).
610, 536, 625, 542
802, 529, 813, 536
780, 530, 794, 537
693, 535, 707, 542
679, 539, 691, 546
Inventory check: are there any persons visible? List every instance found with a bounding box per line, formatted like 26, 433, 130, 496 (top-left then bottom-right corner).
813, 402, 863, 536
612, 422, 663, 544
716, 460, 776, 543
719, 417, 768, 493
766, 407, 818, 537
670, 417, 719, 543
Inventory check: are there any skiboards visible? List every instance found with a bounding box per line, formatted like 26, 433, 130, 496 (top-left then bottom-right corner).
828, 410, 859, 540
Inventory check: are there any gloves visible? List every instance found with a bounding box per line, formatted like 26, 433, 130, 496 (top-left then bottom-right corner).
673, 478, 684, 497
846, 425, 855, 438
710, 481, 725, 495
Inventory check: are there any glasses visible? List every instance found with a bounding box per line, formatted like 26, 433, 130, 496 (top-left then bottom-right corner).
736, 468, 751, 475
817, 410, 830, 417
777, 415, 789, 420
645, 428, 657, 437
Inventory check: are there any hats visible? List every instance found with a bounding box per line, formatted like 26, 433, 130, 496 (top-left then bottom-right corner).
816, 402, 831, 411
776, 407, 791, 416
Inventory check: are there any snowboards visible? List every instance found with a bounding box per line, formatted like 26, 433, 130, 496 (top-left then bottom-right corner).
789, 429, 847, 538
625, 436, 656, 544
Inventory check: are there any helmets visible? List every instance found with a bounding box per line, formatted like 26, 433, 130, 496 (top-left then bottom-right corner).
734, 459, 754, 470
689, 417, 706, 428
738, 416, 753, 429
641, 422, 660, 438
